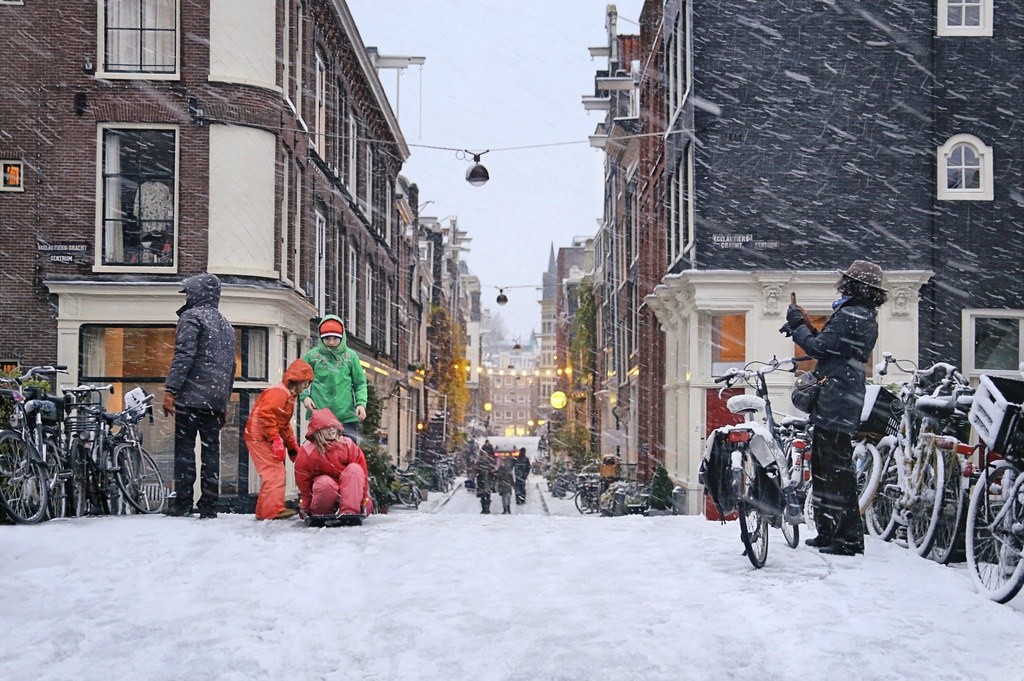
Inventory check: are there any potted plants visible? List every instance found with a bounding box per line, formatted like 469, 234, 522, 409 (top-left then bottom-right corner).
0, 369, 65, 424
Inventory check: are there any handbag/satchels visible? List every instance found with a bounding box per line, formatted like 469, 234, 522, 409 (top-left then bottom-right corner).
696, 420, 787, 517
791, 370, 821, 414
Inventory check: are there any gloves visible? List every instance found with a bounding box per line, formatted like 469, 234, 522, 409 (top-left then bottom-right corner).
298, 500, 309, 519
271, 438, 286, 462
287, 440, 301, 463
162, 391, 175, 416
219, 412, 226, 430
788, 303, 804, 329
360, 497, 373, 517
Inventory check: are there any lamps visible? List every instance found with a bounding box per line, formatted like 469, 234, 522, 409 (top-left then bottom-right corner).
495, 286, 510, 304
512, 337, 522, 353
515, 374, 521, 382
507, 359, 514, 370
464, 150, 491, 186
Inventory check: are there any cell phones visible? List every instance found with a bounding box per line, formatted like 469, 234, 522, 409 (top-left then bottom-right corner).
791, 292, 796, 305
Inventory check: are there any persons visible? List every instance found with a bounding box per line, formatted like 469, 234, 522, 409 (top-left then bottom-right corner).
779, 258, 890, 556
161, 273, 236, 519
464, 440, 531, 514
294, 408, 373, 526
301, 313, 368, 446
242, 358, 314, 519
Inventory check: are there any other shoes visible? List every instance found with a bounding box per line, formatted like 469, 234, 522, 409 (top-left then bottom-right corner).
819, 546, 851, 555
160, 505, 193, 516
806, 539, 829, 548
255, 508, 297, 520
200, 510, 217, 518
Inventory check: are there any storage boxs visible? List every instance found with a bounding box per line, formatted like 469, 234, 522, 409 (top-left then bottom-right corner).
860, 385, 901, 436
967, 375, 1024, 454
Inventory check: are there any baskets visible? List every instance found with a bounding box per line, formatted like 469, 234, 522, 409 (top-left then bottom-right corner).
859, 385, 904, 437
939, 409, 971, 440
969, 375, 1024, 458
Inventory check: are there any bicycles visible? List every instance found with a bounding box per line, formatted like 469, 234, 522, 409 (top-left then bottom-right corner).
763, 353, 1024, 604
0, 365, 165, 526
391, 453, 466, 510
532, 451, 640, 515
712, 356, 813, 568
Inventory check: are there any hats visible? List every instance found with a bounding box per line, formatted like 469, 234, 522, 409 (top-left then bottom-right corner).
178, 284, 187, 294
837, 260, 888, 292
320, 320, 342, 339
